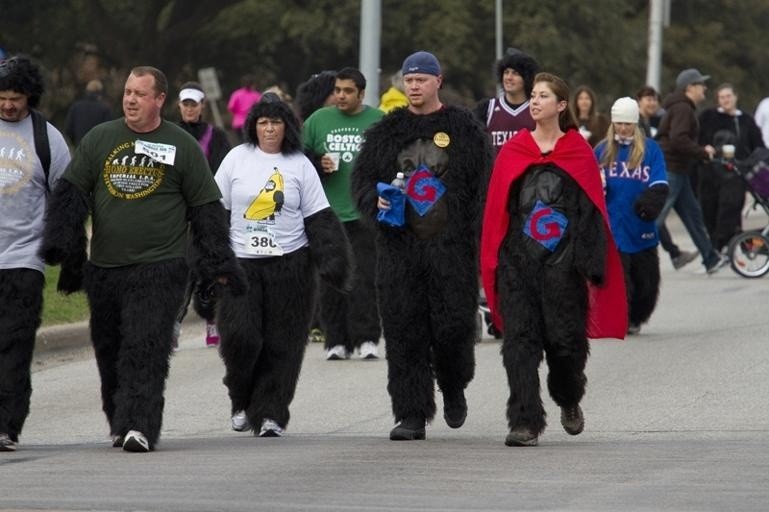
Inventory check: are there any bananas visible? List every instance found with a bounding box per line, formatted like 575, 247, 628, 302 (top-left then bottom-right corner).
242, 165, 285, 221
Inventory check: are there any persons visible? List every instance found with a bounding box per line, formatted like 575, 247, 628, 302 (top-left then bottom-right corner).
350, 51, 498, 441
480, 72, 626, 447
63, 80, 113, 149
38, 67, 250, 453
378, 69, 410, 114
295, 70, 337, 342
0, 54, 88, 450
472, 48, 769, 339
302, 66, 387, 361
188, 92, 355, 439
170, 81, 231, 349
226, 76, 260, 134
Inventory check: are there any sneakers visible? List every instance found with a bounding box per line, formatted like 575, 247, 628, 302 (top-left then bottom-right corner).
390, 423, 425, 441
504, 426, 539, 447
123, 430, 150, 452
444, 387, 466, 428
231, 410, 252, 431
309, 329, 325, 343
254, 418, 285, 437
326, 345, 351, 359
111, 433, 123, 446
0, 433, 18, 452
560, 404, 584, 435
359, 340, 379, 360
206, 323, 220, 348
672, 249, 700, 270
169, 336, 180, 352
707, 255, 730, 274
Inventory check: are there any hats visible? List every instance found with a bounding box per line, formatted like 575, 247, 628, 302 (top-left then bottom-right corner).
402, 51, 441, 77
179, 89, 205, 103
676, 68, 711, 89
611, 96, 639, 123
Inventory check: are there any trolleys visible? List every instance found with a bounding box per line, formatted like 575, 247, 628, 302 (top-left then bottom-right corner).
703, 143, 769, 280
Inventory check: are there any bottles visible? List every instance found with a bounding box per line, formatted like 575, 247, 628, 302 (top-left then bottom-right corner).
390, 171, 409, 191
